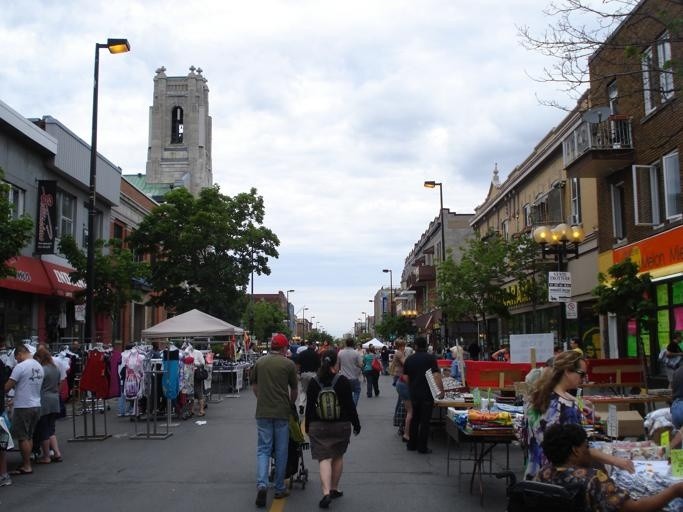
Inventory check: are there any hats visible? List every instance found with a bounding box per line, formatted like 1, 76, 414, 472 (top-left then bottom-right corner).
271, 334, 288, 347
450, 346, 463, 353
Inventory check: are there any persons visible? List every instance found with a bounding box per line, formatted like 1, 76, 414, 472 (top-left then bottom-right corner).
248, 341, 256, 353
525, 356, 556, 395
335, 338, 366, 408
37, 343, 48, 353
664, 332, 683, 395
381, 346, 397, 376
0, 358, 13, 486
363, 348, 380, 397
458, 342, 470, 360
469, 340, 483, 360
369, 344, 374, 348
4, 345, 44, 474
65, 341, 84, 400
296, 340, 309, 354
396, 373, 412, 442
236, 347, 242, 362
525, 351, 636, 482
432, 346, 444, 359
532, 424, 683, 512
402, 338, 445, 453
36, 349, 64, 464
250, 334, 298, 507
194, 344, 208, 409
296, 339, 321, 415
491, 345, 509, 360
188, 342, 205, 417
286, 336, 302, 360
305, 350, 361, 507
392, 339, 413, 435
567, 338, 583, 396
77, 345, 88, 372
118, 342, 134, 416
449, 346, 471, 393
644, 398, 683, 448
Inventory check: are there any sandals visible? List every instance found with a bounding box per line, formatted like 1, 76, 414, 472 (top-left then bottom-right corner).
52, 456, 63, 462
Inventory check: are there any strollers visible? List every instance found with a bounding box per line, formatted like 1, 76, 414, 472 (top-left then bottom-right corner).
269, 400, 311, 491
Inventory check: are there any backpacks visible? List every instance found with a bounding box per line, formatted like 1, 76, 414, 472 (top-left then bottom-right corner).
312, 374, 342, 421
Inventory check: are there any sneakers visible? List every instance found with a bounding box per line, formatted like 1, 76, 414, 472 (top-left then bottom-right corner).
256, 487, 267, 507
275, 490, 290, 499
0, 476, 12, 487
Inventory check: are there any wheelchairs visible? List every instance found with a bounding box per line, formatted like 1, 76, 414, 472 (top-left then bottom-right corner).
496, 469, 591, 512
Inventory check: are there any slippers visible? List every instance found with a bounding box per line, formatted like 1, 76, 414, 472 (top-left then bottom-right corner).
198, 411, 206, 417
10, 468, 32, 475
35, 458, 50, 463
402, 437, 411, 442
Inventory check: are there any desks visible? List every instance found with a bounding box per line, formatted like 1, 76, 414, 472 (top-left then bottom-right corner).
578, 394, 671, 425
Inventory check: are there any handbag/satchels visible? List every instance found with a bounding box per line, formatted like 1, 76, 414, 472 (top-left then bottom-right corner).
196, 365, 208, 381
372, 354, 382, 372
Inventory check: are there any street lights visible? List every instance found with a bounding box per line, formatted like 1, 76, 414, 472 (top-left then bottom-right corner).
287, 290, 294, 334
303, 308, 308, 340
311, 317, 315, 333
401, 309, 418, 332
316, 322, 320, 335
382, 270, 393, 318
369, 300, 375, 325
362, 312, 366, 334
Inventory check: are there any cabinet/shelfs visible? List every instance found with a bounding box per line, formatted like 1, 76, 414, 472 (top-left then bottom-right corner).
212, 355, 259, 394
446, 407, 516, 507
433, 393, 478, 407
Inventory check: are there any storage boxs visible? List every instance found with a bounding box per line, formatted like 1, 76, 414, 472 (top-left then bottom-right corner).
607, 410, 644, 438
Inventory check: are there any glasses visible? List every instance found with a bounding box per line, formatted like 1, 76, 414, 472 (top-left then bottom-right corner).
575, 370, 587, 377
580, 442, 592, 447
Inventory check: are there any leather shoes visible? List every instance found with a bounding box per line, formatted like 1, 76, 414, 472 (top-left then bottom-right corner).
320, 495, 330, 506
418, 448, 432, 453
407, 444, 418, 450
330, 490, 344, 497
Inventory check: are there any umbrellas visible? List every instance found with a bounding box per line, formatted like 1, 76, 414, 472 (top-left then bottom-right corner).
140, 308, 247, 340
361, 337, 386, 348
